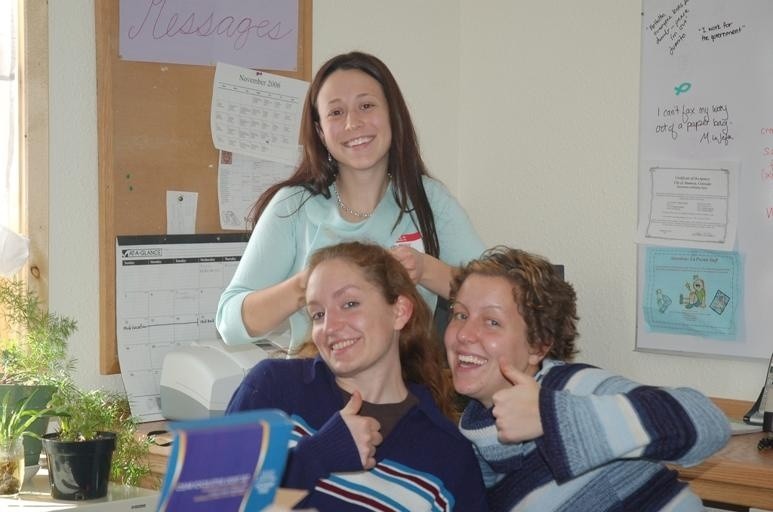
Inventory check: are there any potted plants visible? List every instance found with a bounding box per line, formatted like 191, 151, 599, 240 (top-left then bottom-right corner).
0, 274, 159, 502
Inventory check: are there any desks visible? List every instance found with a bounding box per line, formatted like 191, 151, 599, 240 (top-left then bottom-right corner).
661, 392, 773, 512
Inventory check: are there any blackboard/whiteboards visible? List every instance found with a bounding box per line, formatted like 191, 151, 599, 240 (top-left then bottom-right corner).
633, 2, 773, 363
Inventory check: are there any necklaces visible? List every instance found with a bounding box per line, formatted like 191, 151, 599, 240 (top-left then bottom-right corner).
332, 172, 392, 217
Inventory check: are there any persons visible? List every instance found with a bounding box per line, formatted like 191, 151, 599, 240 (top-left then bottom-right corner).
214, 51, 486, 360
223, 241, 489, 511
444, 244, 732, 511
